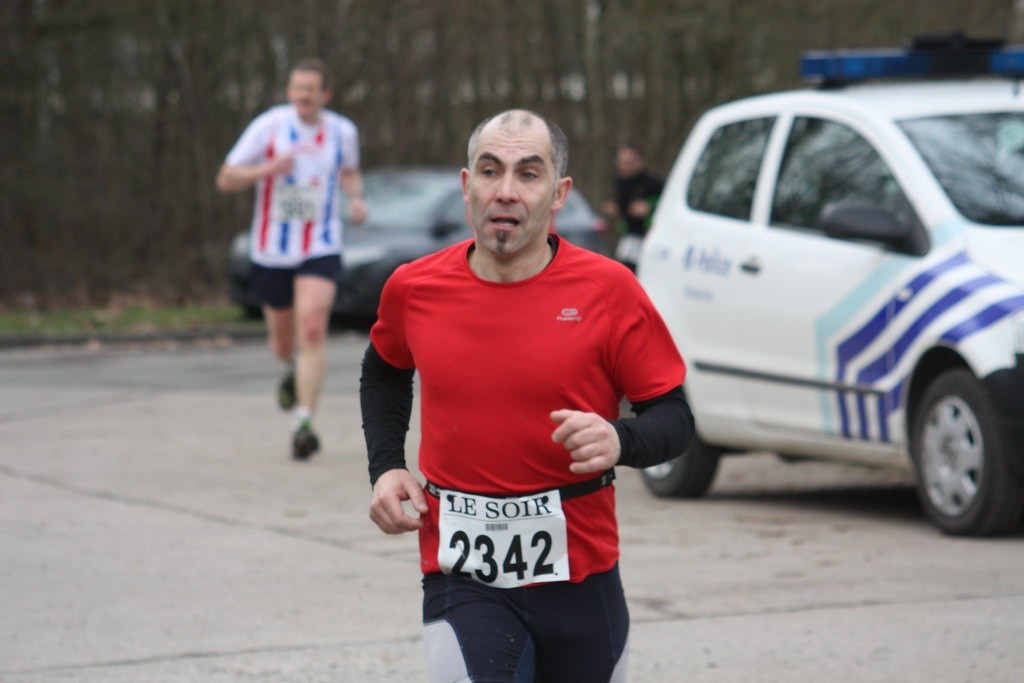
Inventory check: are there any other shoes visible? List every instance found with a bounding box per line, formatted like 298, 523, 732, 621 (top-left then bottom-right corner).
280, 375, 294, 408
293, 421, 318, 458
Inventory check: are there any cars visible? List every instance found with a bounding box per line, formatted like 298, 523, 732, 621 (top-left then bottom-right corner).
224, 169, 608, 320
631, 32, 1023, 536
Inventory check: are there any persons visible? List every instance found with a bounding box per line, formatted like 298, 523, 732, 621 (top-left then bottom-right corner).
603, 140, 668, 273
215, 57, 368, 458
357, 111, 698, 683
